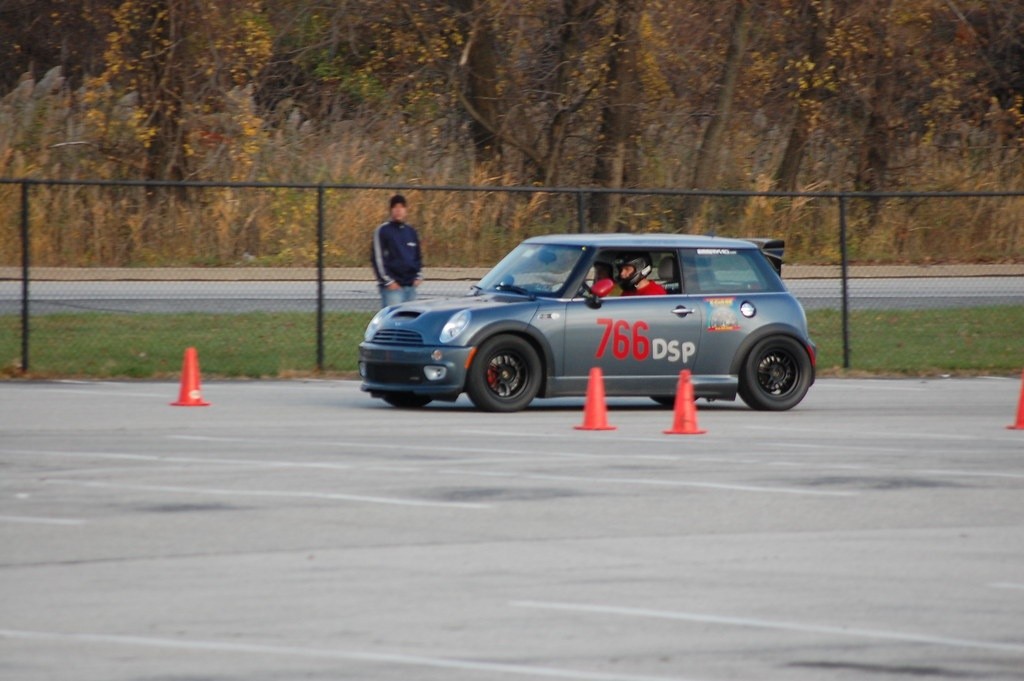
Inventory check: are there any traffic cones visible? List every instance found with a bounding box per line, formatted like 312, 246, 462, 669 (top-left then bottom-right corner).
1003, 368, 1024, 431
167, 346, 213, 407
662, 367, 708, 435
573, 363, 616, 431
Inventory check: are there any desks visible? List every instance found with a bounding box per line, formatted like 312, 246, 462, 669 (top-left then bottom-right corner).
659, 255, 680, 293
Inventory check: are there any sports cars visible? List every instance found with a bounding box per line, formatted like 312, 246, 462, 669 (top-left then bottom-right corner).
358, 232, 817, 414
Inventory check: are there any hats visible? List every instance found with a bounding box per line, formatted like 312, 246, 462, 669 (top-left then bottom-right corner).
390, 194, 408, 208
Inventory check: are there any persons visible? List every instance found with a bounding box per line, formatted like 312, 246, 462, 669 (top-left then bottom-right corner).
594, 252, 624, 297
617, 250, 666, 297
371, 195, 424, 309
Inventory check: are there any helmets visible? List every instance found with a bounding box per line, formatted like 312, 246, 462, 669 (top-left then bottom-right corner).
593, 249, 616, 269
611, 254, 652, 291
